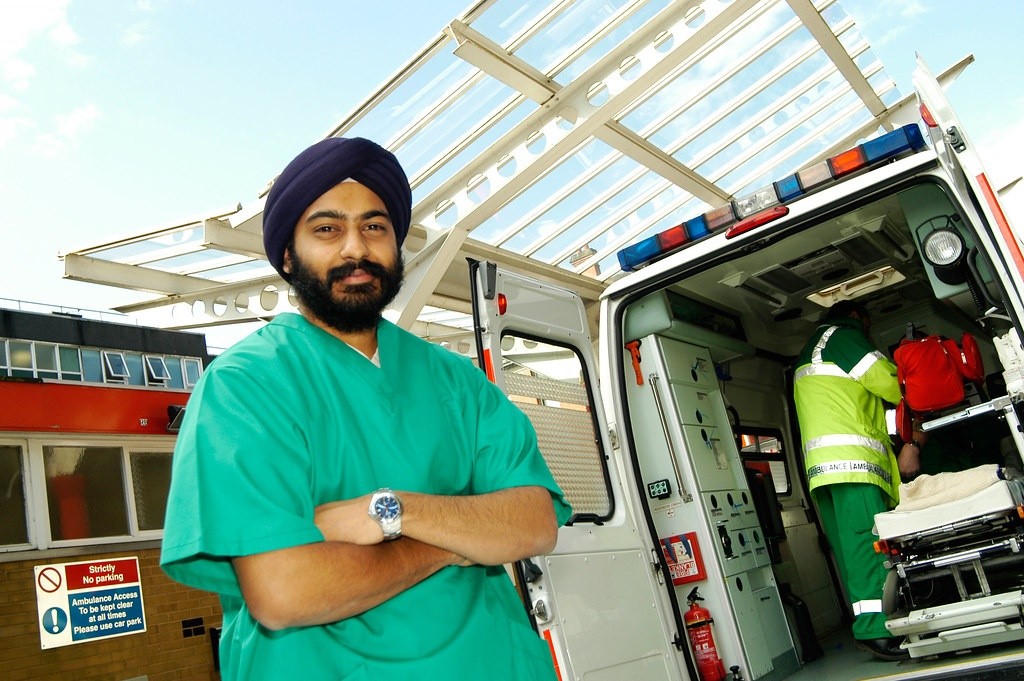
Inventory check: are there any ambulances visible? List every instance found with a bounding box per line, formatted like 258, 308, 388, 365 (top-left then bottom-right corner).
465, 52, 1024, 681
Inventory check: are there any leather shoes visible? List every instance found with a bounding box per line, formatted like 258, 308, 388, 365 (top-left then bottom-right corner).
854, 637, 910, 659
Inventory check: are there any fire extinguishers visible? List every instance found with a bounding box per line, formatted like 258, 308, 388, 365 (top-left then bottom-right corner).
683, 586, 727, 681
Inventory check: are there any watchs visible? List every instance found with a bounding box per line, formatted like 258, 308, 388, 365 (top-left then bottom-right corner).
368, 489, 402, 541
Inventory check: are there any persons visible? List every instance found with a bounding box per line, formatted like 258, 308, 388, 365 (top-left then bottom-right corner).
792, 301, 912, 661
892, 331, 1002, 480
161, 136, 578, 681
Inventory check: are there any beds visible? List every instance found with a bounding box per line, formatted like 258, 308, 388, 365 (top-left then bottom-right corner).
875, 462, 1020, 541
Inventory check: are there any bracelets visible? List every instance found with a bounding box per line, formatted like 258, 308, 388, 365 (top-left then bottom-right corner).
907, 438, 922, 450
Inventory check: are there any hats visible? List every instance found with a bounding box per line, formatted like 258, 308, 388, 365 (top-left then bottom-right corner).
263, 137, 412, 284
